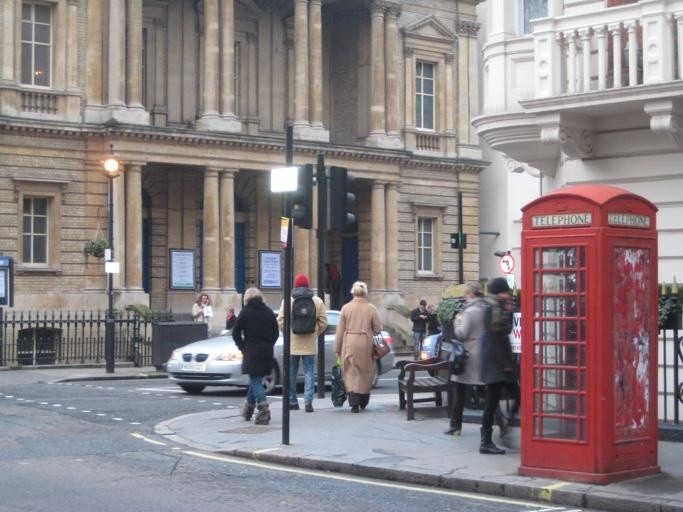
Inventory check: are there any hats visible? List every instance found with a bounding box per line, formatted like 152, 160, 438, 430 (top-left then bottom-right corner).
487, 278, 513, 295
294, 275, 308, 288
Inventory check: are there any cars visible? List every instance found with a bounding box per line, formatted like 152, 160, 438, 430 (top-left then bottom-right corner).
413, 312, 575, 379
167, 309, 395, 394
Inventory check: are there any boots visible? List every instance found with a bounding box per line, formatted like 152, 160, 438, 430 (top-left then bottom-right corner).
493, 407, 510, 437
242, 400, 254, 420
480, 427, 505, 454
254, 402, 270, 425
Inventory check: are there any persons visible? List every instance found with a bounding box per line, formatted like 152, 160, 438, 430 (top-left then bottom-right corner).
275, 274, 329, 412
410, 300, 429, 360
232, 287, 279, 425
426, 305, 441, 336
225, 307, 238, 330
334, 281, 382, 412
443, 281, 509, 438
479, 278, 520, 453
191, 294, 212, 338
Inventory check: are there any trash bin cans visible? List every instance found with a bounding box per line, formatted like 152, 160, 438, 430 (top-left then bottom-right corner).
151, 321, 208, 372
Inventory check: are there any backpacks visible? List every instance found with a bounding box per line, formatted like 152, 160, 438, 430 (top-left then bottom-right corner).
291, 295, 316, 333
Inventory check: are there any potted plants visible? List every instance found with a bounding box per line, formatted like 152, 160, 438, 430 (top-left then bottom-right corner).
437, 299, 465, 342
85, 240, 111, 258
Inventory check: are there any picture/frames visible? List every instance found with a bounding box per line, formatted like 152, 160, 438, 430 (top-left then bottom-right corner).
259, 250, 282, 289
169, 248, 195, 290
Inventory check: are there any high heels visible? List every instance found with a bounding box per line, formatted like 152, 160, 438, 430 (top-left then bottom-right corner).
445, 427, 462, 435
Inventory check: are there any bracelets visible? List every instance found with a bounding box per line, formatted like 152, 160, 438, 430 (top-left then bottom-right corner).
504, 302, 513, 304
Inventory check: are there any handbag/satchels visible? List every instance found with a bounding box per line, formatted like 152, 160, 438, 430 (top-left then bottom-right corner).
332, 365, 347, 407
372, 340, 390, 360
441, 339, 465, 375
203, 306, 213, 318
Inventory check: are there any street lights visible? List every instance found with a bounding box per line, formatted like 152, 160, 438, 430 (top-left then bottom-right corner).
98, 144, 123, 374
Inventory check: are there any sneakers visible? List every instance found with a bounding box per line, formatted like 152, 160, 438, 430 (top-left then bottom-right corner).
351, 406, 359, 413
289, 402, 299, 410
360, 405, 365, 409
306, 402, 313, 412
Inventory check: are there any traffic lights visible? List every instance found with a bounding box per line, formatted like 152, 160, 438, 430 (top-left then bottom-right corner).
291, 164, 312, 229
329, 166, 355, 232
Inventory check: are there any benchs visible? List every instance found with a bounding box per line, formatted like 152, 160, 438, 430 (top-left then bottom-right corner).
396, 339, 456, 421
